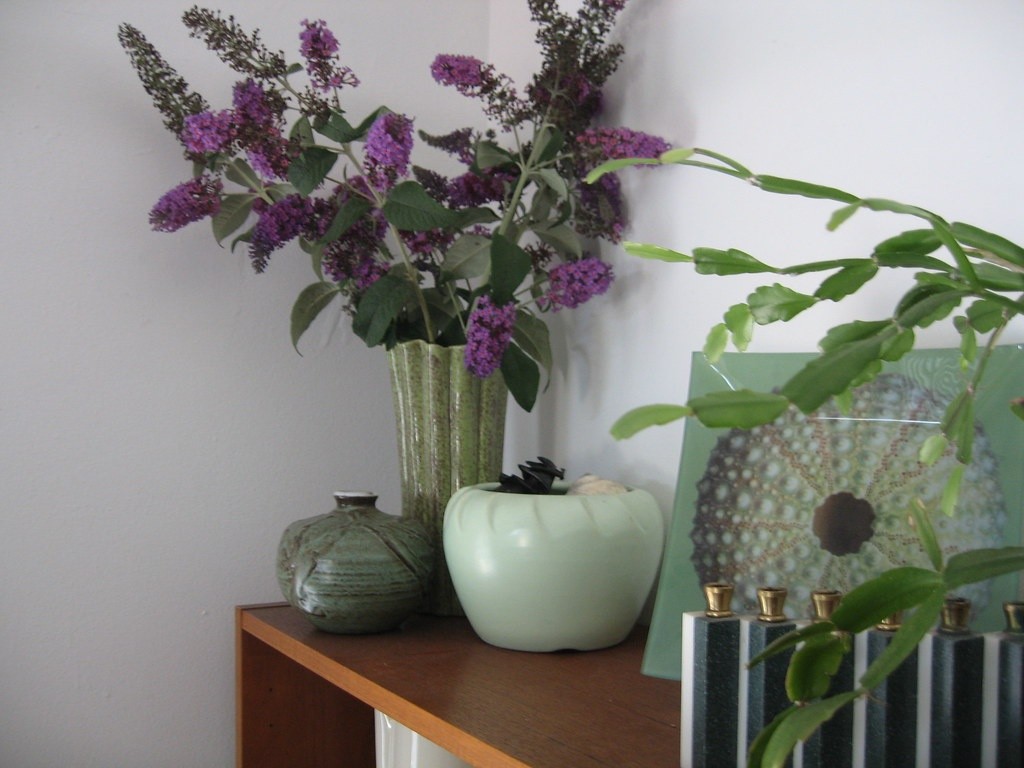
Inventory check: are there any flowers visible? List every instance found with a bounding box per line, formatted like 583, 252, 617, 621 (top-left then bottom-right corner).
117, 0, 675, 415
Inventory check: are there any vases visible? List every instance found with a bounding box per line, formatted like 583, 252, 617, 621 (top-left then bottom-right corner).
277, 489, 436, 635
378, 339, 512, 621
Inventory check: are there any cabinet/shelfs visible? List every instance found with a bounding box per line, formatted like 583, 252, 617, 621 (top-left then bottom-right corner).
231, 605, 686, 767
